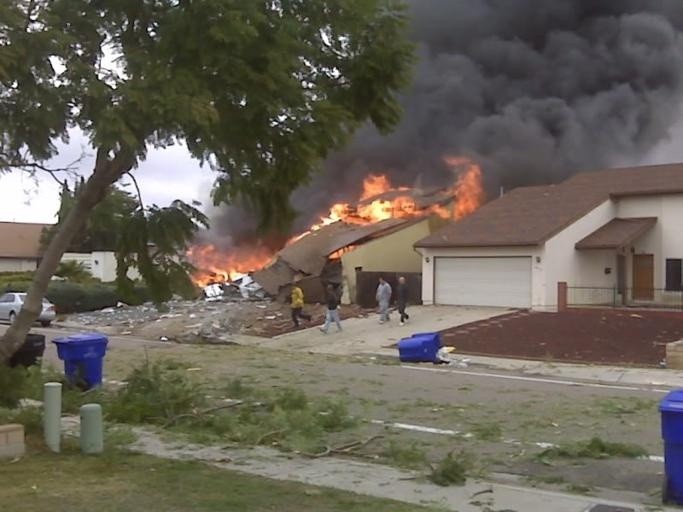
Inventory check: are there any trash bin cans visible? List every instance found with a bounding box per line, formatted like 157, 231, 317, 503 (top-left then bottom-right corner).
399, 332, 441, 362
658, 391, 683, 504
9, 334, 107, 390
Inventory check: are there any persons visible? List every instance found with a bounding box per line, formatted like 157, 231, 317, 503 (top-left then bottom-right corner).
375, 275, 411, 325
288, 280, 312, 328
319, 284, 343, 333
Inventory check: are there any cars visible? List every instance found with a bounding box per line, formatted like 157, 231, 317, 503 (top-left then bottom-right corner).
0, 292, 57, 326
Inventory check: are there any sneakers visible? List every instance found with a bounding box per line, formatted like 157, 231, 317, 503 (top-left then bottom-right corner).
378, 315, 410, 326
319, 327, 343, 334
